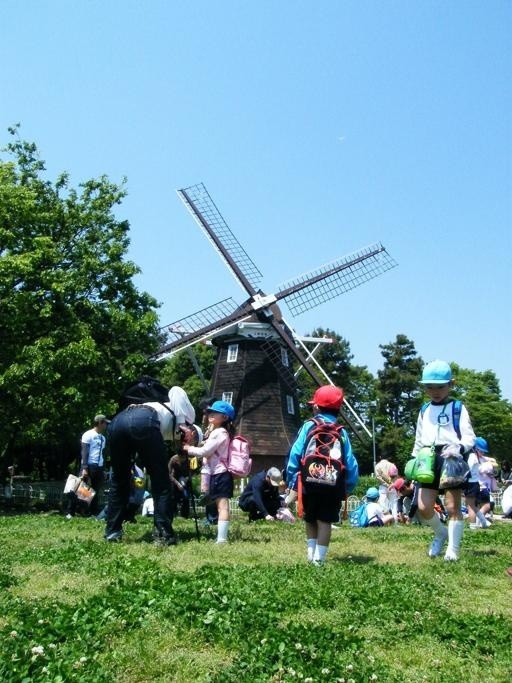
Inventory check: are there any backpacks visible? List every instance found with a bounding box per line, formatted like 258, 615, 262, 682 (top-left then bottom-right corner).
299, 423, 346, 487
228, 434, 253, 478
388, 464, 399, 477
276, 507, 295, 523
350, 503, 369, 528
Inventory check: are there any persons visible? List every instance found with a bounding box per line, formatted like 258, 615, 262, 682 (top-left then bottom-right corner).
33, 360, 508, 569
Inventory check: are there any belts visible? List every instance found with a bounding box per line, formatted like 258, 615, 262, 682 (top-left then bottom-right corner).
128, 405, 155, 412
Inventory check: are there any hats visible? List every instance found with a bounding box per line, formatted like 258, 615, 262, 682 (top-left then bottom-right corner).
306, 385, 344, 410
143, 491, 150, 498
192, 423, 203, 447
420, 359, 452, 386
94, 415, 112, 424
366, 487, 380, 499
393, 477, 404, 490
266, 466, 283, 487
206, 400, 235, 420
475, 436, 489, 453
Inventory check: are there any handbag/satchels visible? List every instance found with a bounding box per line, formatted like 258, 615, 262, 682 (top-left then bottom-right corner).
79, 463, 98, 474
63, 473, 96, 508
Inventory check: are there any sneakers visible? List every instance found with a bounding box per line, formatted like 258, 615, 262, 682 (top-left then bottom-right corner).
469, 520, 491, 529
476, 511, 487, 528
428, 527, 448, 557
66, 514, 73, 519
444, 550, 461, 562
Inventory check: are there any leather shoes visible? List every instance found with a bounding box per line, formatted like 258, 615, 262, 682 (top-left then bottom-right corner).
161, 531, 178, 545
104, 531, 122, 543
